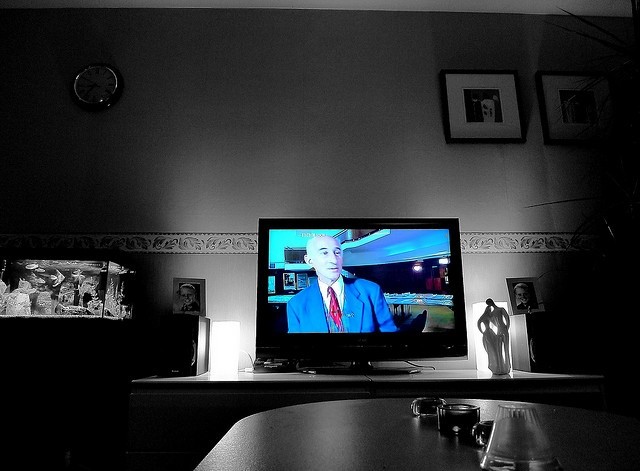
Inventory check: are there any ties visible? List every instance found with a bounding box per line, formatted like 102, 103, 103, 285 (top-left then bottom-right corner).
326, 285, 342, 331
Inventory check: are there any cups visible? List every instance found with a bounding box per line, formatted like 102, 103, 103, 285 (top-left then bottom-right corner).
480, 403, 563, 471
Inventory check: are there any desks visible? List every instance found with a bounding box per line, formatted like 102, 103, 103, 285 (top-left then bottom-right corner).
190, 396, 639, 471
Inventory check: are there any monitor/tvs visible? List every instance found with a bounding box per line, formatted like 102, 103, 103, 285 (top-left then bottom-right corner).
255, 216, 468, 374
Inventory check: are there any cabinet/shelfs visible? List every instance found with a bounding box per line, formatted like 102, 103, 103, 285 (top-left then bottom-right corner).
128, 362, 605, 468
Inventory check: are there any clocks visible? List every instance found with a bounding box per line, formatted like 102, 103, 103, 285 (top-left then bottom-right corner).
68, 60, 122, 111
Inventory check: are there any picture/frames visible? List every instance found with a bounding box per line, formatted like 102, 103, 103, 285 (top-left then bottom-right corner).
172, 278, 206, 316
506, 276, 546, 313
535, 69, 611, 146
439, 68, 528, 144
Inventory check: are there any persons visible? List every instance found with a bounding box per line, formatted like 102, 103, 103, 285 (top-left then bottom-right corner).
286, 234, 428, 333
513, 282, 539, 310
477, 298, 510, 374
178, 283, 200, 311
